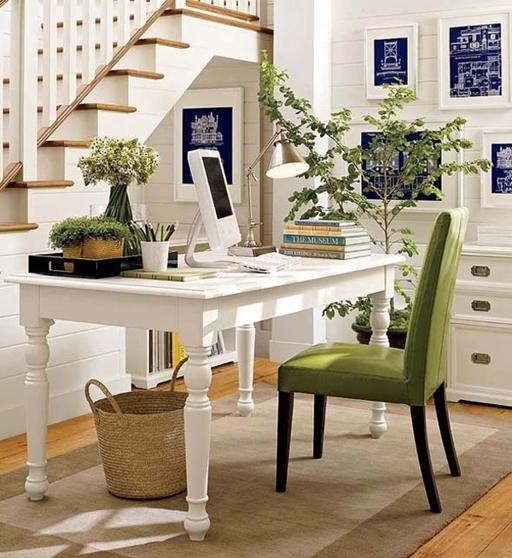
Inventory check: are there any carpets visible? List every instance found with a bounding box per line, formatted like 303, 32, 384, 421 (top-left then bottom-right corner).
0, 381, 512, 558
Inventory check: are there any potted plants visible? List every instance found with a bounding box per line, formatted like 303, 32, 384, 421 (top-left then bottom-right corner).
259, 50, 496, 350
45, 217, 126, 259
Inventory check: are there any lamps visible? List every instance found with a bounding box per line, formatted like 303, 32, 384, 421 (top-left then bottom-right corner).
229, 121, 309, 258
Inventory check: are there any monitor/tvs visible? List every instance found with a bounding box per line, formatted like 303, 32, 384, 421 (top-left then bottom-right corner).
184, 149, 243, 271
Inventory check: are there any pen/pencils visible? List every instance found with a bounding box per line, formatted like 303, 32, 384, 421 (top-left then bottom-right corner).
129, 220, 176, 242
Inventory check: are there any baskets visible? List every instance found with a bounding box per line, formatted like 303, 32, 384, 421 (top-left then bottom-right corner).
85, 357, 190, 500
61, 238, 122, 259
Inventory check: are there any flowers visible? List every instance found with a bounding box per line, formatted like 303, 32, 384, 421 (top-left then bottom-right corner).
77, 136, 161, 221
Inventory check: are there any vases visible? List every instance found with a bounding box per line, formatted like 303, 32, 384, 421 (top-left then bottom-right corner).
85, 204, 146, 231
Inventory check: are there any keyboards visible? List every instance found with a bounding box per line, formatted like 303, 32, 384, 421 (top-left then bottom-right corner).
242, 252, 301, 273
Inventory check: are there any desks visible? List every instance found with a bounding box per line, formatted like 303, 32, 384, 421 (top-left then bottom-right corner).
5, 251, 408, 541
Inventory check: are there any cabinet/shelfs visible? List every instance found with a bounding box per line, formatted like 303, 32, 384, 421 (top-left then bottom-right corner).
447, 247, 512, 412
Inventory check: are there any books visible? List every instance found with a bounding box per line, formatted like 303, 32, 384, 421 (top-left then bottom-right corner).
279, 218, 371, 259
120, 269, 217, 282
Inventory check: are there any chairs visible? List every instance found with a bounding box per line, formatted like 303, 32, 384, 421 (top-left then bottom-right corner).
276, 204, 470, 514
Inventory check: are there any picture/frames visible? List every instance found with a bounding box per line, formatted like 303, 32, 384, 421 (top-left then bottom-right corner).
480, 127, 512, 209
438, 11, 512, 111
170, 89, 244, 203
336, 121, 464, 215
362, 23, 421, 102
